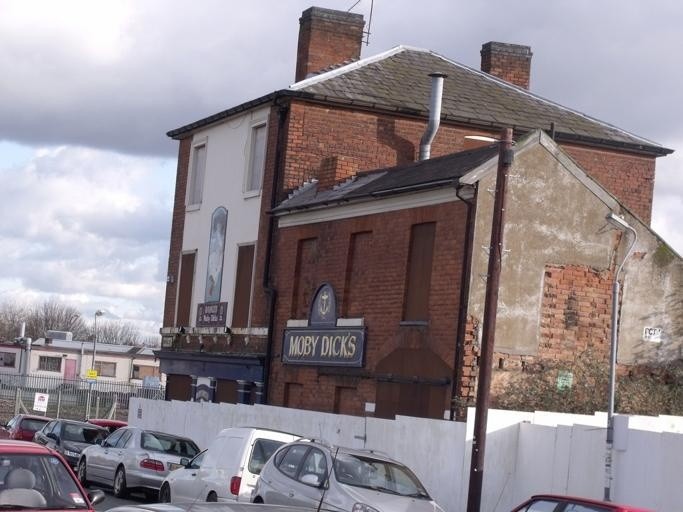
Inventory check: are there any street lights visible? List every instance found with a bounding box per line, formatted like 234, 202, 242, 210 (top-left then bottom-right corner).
84, 309, 105, 420
604, 212, 638, 500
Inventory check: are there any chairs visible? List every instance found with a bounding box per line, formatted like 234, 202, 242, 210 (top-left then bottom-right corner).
0, 466, 46, 507
